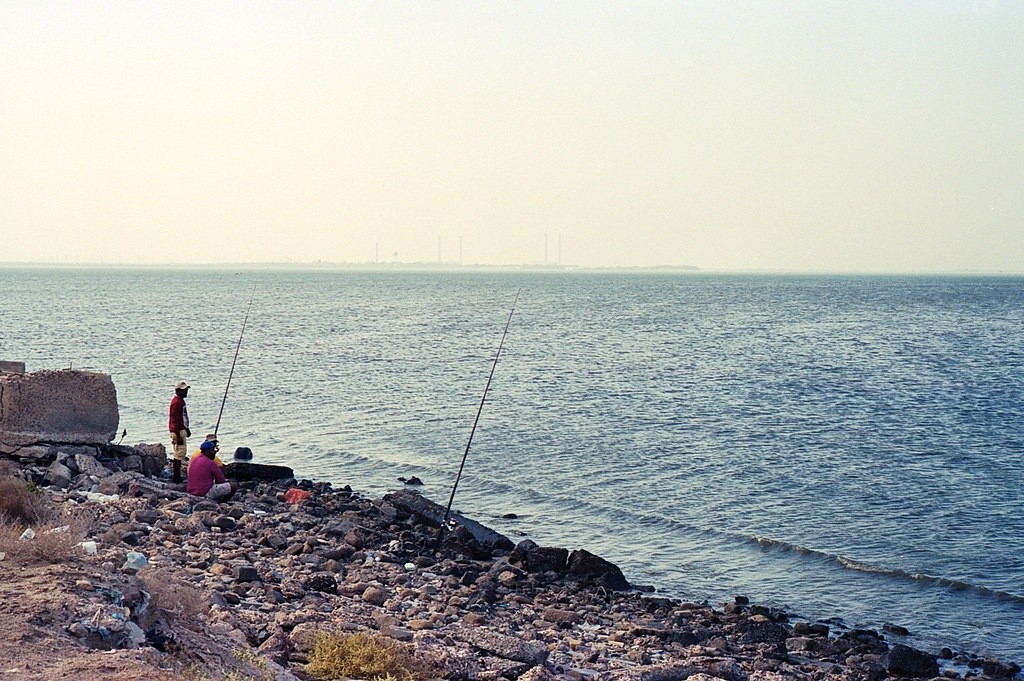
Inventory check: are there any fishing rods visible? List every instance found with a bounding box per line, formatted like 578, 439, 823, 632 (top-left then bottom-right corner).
212, 283, 257, 453
429, 287, 522, 559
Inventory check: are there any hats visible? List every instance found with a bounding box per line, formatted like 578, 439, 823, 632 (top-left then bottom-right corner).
200, 441, 214, 449
175, 381, 191, 390
206, 434, 219, 443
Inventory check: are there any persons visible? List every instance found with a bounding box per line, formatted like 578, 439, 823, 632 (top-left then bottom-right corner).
187, 434, 224, 476
186, 441, 237, 504
168, 382, 191, 485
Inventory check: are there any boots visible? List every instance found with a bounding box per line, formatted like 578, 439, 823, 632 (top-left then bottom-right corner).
172, 458, 183, 483
